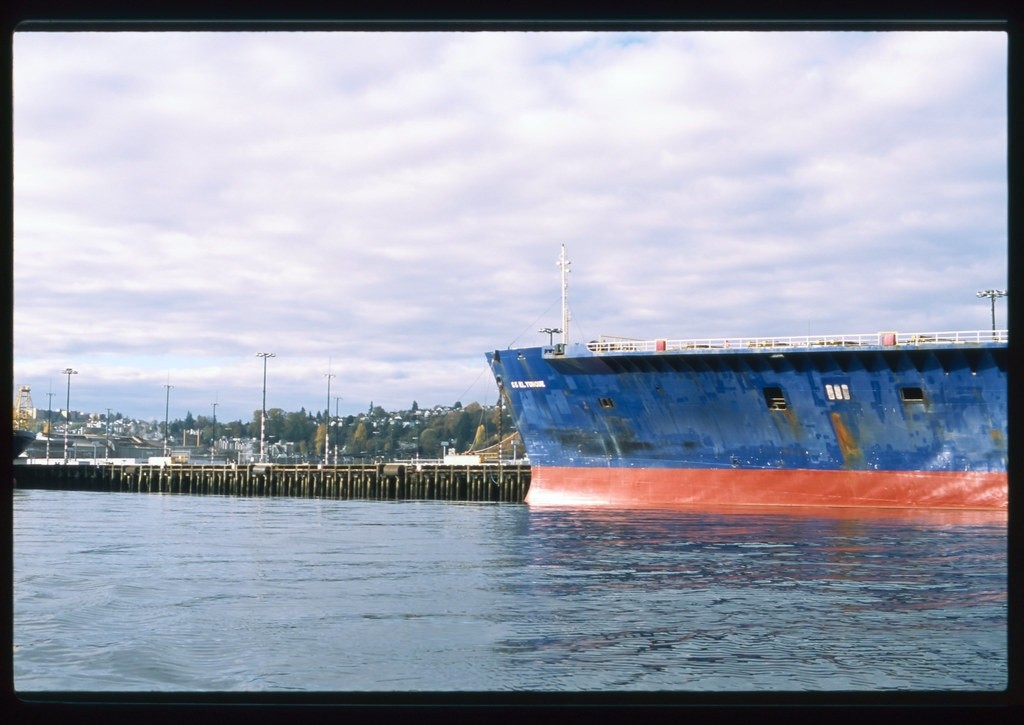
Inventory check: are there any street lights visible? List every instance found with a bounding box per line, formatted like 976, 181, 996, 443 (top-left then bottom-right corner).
60, 368, 78, 463
162, 384, 175, 457
256, 352, 276, 462
322, 372, 337, 466
209, 402, 220, 464
333, 396, 343, 465
46, 392, 57, 465
977, 289, 1009, 340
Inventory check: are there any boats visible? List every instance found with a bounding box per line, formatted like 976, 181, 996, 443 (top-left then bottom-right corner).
481, 240, 1009, 510
12, 383, 38, 459
